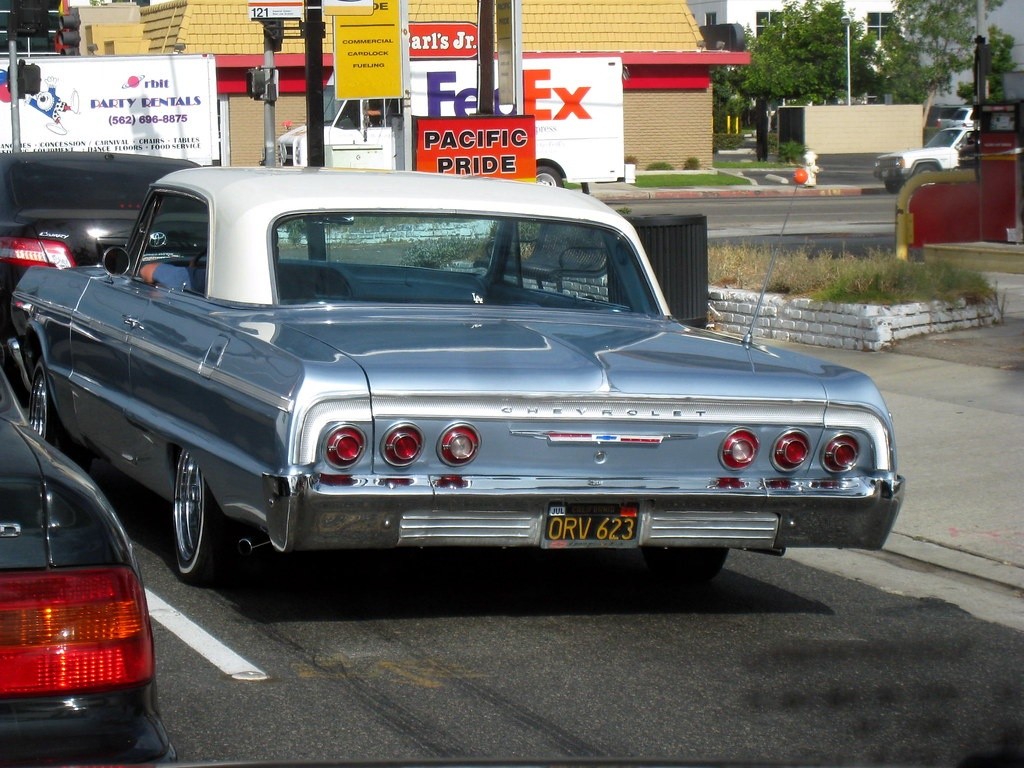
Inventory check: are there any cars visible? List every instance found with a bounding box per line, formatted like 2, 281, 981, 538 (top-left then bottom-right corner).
0, 366, 178, 768
937, 106, 977, 129
7, 164, 906, 586
1, 148, 278, 382
872, 127, 981, 196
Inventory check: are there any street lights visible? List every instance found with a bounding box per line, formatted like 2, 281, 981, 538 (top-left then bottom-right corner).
840, 16, 853, 106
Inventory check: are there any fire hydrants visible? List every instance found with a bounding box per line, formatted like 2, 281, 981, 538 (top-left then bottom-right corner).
801, 150, 820, 187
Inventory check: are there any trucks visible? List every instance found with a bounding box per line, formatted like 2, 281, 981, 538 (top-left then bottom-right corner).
0, 49, 221, 167
262, 55, 636, 189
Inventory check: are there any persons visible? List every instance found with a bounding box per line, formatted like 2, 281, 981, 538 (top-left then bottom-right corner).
364, 99, 390, 120
139, 231, 278, 292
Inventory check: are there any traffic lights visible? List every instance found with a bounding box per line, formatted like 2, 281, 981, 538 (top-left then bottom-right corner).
246, 67, 266, 100
60, 7, 79, 55
